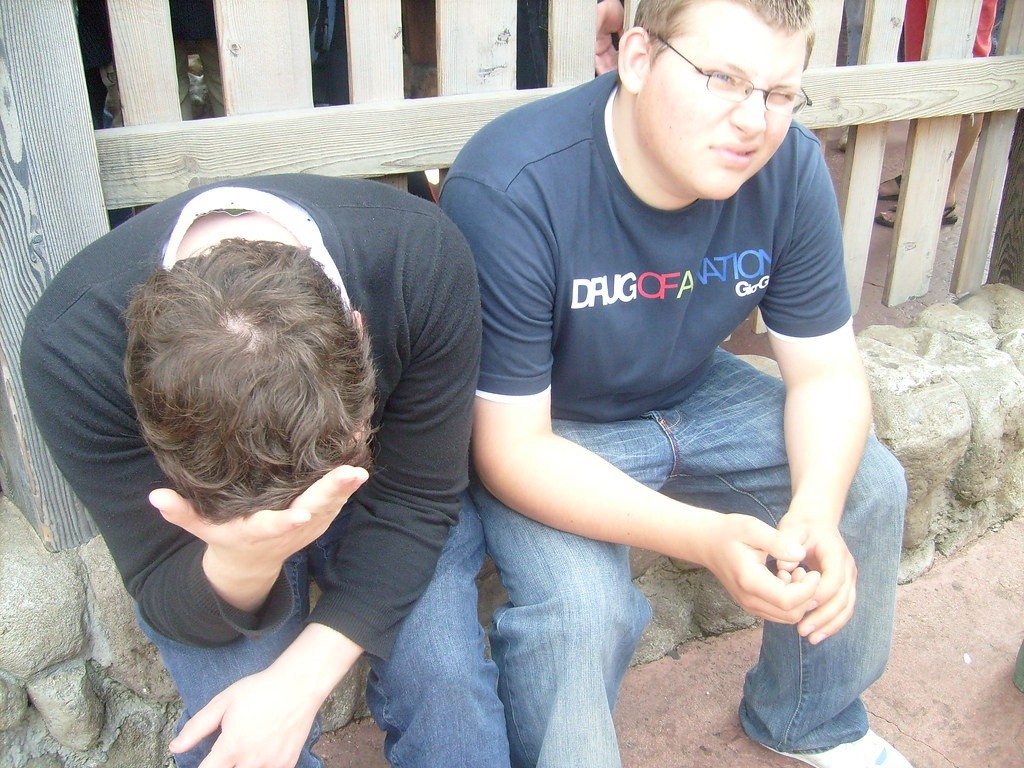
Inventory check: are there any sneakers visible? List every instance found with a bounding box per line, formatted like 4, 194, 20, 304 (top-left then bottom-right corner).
758, 728, 916, 768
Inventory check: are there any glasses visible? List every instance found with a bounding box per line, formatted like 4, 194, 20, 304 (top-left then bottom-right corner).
646, 28, 813, 116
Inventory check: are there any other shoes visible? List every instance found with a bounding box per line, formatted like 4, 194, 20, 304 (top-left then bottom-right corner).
838, 126, 849, 153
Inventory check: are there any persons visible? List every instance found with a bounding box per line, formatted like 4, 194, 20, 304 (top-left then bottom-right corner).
440, 0, 917, 768
73, 0, 549, 227
20, 170, 515, 768
839, 1, 1003, 226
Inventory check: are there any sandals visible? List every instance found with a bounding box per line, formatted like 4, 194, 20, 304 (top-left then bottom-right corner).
874, 201, 958, 224
877, 175, 902, 201
